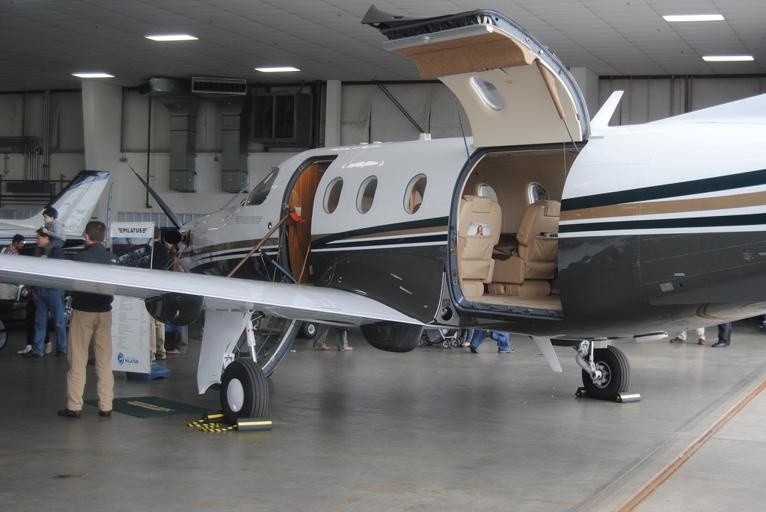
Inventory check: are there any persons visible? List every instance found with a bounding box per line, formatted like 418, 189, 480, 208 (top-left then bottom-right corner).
313, 323, 353, 352
461, 329, 475, 347
0, 208, 188, 361
670, 327, 706, 344
712, 322, 731, 347
469, 330, 515, 352
57, 221, 113, 417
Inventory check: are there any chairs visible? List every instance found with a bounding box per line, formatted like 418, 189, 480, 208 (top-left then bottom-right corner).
457, 194, 502, 297
488, 200, 561, 296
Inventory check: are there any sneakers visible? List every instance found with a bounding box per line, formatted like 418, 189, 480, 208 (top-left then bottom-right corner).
470, 345, 479, 353
319, 344, 352, 351
671, 337, 728, 347
17, 342, 67, 357
58, 408, 82, 417
499, 348, 516, 353
151, 347, 183, 361
99, 410, 112, 416
